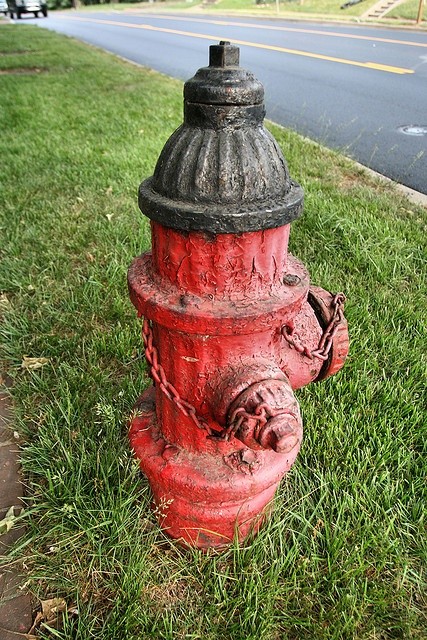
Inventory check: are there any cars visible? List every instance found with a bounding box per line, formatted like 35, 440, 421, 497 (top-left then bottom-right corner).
0, 1, 7, 14
6, 0, 47, 20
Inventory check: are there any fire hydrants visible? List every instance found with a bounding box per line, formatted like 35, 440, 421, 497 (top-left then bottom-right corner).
126, 41, 349, 552
